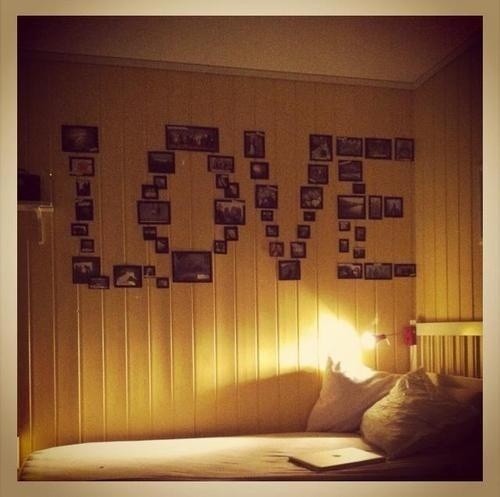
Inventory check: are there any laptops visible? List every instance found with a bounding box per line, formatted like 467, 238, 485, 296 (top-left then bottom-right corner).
288, 447, 386, 471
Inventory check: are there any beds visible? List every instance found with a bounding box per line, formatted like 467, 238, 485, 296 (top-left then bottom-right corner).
18, 313, 482, 481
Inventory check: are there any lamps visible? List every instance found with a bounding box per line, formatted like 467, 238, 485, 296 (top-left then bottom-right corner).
357, 321, 417, 350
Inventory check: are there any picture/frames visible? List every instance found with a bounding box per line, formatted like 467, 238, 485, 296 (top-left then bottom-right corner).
61, 124, 99, 153
75, 199, 94, 221
142, 175, 168, 200
76, 178, 91, 197
213, 240, 227, 254
278, 260, 301, 280
166, 124, 221, 153
261, 210, 274, 221
81, 238, 95, 253
113, 264, 143, 287
353, 183, 366, 194
365, 137, 393, 160
265, 225, 279, 236
394, 137, 415, 161
337, 263, 363, 280
155, 237, 169, 253
309, 133, 333, 162
207, 155, 235, 173
224, 226, 239, 241
297, 224, 311, 238
269, 242, 285, 257
308, 164, 329, 184
385, 197, 403, 217
369, 194, 383, 220
337, 195, 367, 220
214, 199, 246, 226
215, 174, 241, 198
354, 249, 366, 258
394, 263, 416, 277
337, 136, 363, 157
143, 226, 157, 240
355, 226, 366, 241
71, 257, 109, 287
303, 211, 317, 221
364, 262, 393, 280
300, 186, 323, 209
69, 156, 95, 177
137, 200, 171, 225
290, 241, 307, 259
338, 160, 363, 181
250, 162, 270, 179
339, 220, 351, 232
244, 130, 265, 158
172, 250, 213, 282
338, 239, 349, 253
148, 151, 176, 173
71, 223, 88, 236
143, 265, 155, 278
255, 184, 278, 209
156, 276, 170, 287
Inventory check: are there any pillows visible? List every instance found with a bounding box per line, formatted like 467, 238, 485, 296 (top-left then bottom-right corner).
304, 350, 401, 436
359, 365, 466, 456
